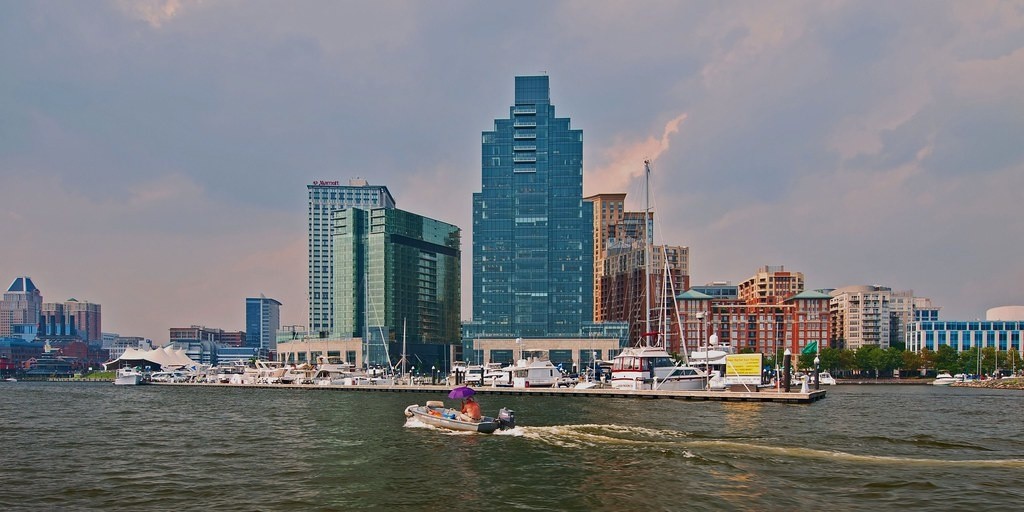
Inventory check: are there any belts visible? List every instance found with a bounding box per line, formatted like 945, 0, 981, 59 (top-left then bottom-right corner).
470, 417, 482, 420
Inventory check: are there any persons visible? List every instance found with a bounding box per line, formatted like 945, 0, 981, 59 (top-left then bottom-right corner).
459, 397, 481, 422
804, 373, 809, 389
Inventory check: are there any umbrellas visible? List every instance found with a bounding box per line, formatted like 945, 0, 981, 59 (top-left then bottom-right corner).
448, 387, 474, 400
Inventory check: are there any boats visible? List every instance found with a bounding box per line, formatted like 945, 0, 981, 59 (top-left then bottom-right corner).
112, 157, 837, 391
404, 400, 517, 435
932, 341, 1024, 386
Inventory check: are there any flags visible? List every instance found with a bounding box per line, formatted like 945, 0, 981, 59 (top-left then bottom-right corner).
802, 341, 817, 354
